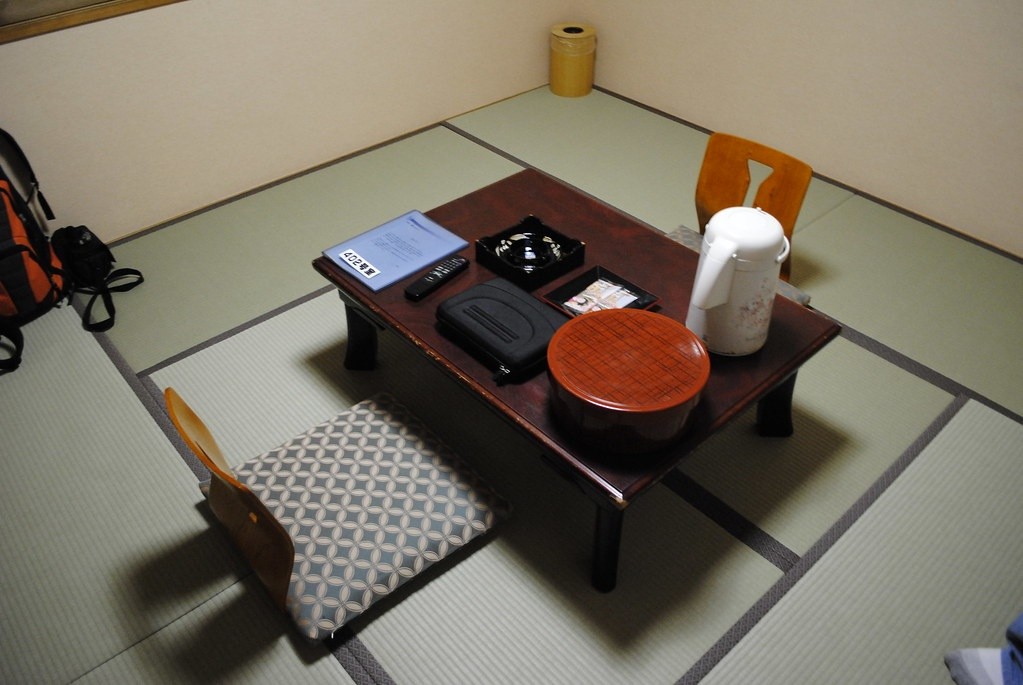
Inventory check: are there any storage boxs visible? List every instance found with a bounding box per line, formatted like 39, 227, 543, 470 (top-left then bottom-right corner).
475, 213, 587, 291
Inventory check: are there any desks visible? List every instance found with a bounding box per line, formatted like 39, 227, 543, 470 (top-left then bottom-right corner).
312, 166, 842, 593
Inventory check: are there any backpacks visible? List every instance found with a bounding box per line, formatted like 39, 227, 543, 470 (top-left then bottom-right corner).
0, 127, 64, 371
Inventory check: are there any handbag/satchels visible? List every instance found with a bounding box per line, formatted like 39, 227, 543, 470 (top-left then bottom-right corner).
51, 224, 144, 331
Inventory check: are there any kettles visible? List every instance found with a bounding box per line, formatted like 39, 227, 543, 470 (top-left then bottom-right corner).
684, 206, 790, 357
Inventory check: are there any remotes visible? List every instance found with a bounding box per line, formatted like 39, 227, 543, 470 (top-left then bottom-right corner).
404, 254, 470, 302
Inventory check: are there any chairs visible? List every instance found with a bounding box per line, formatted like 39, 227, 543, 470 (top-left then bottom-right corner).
663, 130, 816, 311
164, 387, 512, 652
